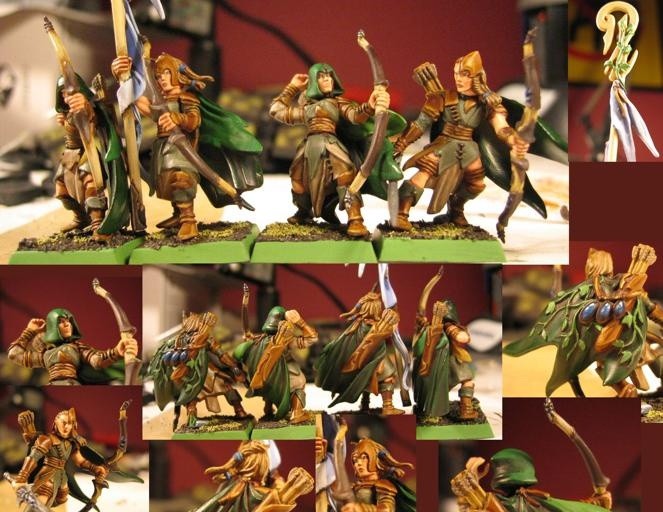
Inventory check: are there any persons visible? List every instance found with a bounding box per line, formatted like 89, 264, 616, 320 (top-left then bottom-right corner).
4, 44, 663, 512
